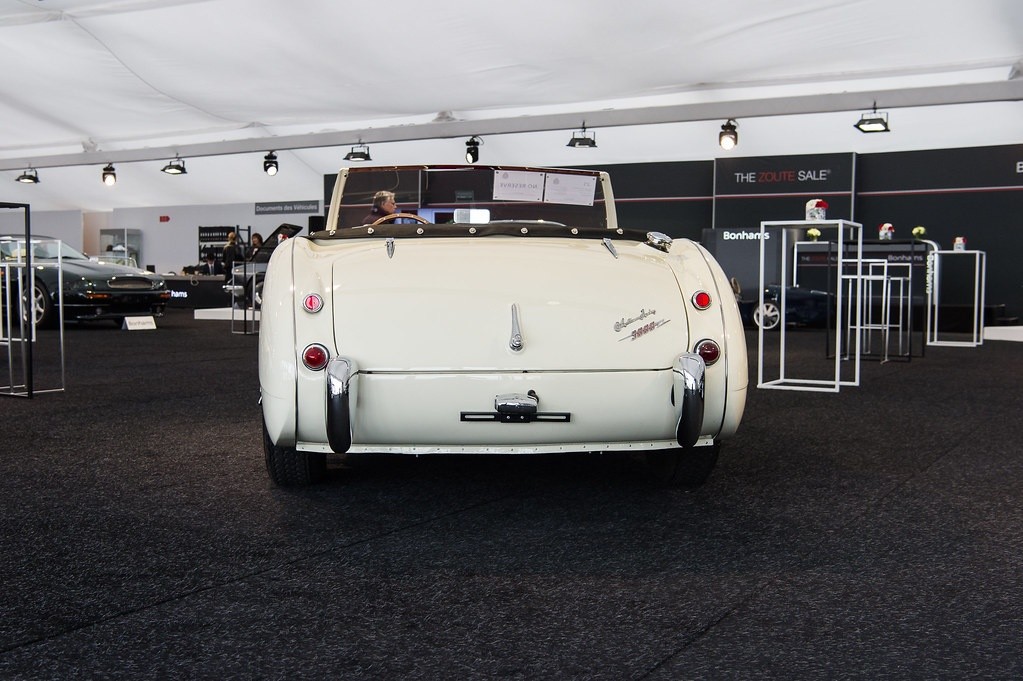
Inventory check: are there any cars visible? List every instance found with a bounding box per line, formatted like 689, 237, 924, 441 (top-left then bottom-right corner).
0, 234, 173, 330
257, 163, 748, 490
223, 224, 302, 309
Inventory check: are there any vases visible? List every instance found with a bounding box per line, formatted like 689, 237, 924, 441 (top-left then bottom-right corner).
915, 233, 922, 239
811, 236, 818, 242
879, 231, 893, 241
953, 243, 966, 250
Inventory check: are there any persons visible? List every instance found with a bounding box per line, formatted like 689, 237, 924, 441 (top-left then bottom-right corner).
245, 233, 263, 259
222, 232, 249, 310
203, 253, 221, 276
361, 191, 396, 225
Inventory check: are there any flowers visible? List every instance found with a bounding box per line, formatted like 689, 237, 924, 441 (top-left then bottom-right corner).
806, 229, 822, 239
911, 226, 926, 237
952, 237, 968, 245
877, 222, 895, 233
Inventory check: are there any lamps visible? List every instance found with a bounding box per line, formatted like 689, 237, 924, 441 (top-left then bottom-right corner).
718, 120, 739, 150
567, 131, 598, 148
102, 163, 116, 187
264, 150, 279, 176
853, 111, 891, 134
161, 157, 187, 175
14, 168, 41, 184
343, 144, 372, 161
466, 135, 479, 163
